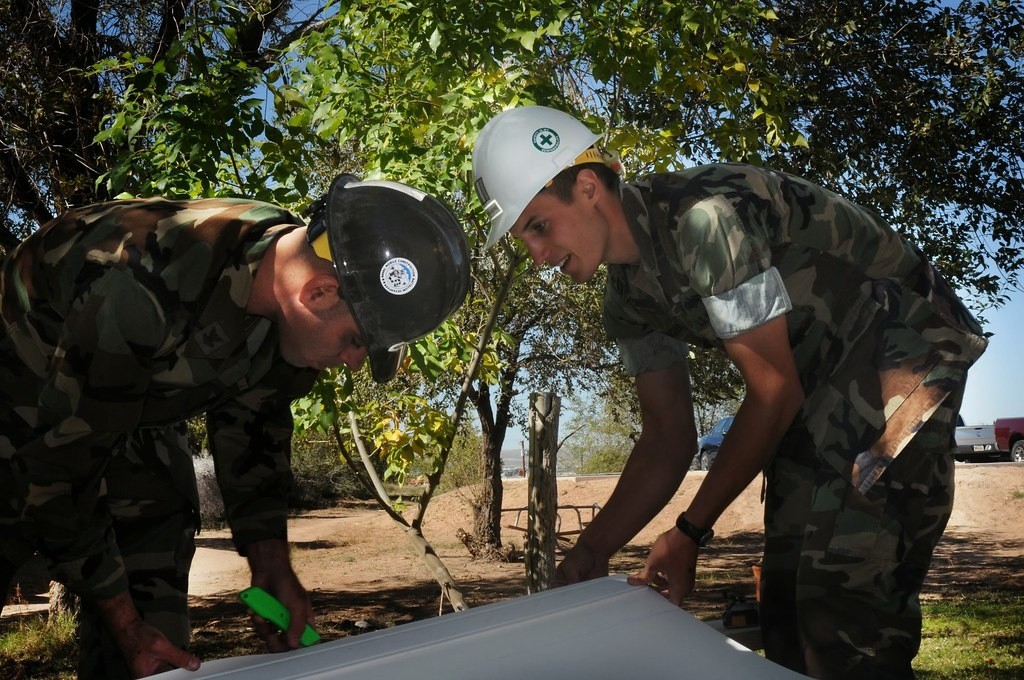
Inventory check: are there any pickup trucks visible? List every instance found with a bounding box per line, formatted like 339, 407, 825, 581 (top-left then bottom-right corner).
995, 416, 1024, 463
952, 415, 1010, 462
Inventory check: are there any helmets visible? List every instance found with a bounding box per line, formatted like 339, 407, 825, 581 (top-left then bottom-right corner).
323, 173, 471, 383
473, 106, 602, 250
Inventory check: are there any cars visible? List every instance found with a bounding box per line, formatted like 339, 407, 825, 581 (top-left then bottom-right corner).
697, 416, 734, 471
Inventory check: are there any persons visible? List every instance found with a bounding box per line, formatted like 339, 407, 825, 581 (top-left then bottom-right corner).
469, 104, 988, 680
0, 173, 469, 680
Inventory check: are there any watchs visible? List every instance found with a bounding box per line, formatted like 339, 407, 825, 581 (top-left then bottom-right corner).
677, 511, 715, 545
